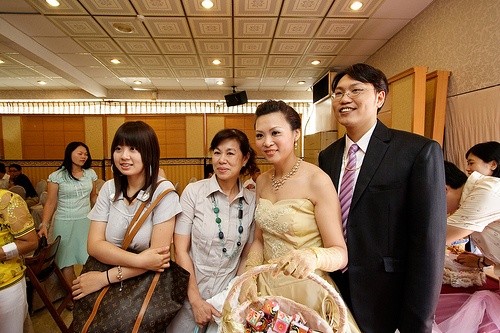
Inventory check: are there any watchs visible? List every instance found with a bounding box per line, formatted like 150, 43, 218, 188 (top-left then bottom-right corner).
480, 257, 490, 267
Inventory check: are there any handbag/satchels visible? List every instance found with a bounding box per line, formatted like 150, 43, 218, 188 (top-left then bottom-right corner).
69, 179, 191, 333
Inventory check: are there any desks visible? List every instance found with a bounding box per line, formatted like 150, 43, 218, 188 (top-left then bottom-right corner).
434, 275, 500, 333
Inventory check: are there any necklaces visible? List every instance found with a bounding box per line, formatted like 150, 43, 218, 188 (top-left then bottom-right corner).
212, 193, 243, 258
271, 157, 302, 190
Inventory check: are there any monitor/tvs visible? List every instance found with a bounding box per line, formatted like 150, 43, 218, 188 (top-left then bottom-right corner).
312, 71, 331, 103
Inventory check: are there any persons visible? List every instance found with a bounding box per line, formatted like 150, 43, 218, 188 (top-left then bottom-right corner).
224, 100, 360, 333
457, 141, 500, 268
9, 164, 39, 207
166, 128, 260, 333
0, 163, 10, 189
38, 142, 98, 310
318, 63, 447, 333
0, 189, 39, 333
443, 160, 500, 264
206, 164, 214, 179
72, 121, 181, 301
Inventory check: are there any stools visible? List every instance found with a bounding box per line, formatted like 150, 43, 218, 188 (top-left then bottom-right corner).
25, 235, 75, 333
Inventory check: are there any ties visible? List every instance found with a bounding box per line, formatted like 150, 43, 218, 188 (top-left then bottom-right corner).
339, 144, 360, 274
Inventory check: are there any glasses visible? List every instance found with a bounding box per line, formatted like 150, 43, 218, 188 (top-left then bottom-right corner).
331, 91, 361, 101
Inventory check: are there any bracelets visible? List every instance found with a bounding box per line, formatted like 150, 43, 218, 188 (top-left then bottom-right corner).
117, 266, 123, 280
107, 269, 110, 284
478, 258, 480, 268
2, 242, 19, 260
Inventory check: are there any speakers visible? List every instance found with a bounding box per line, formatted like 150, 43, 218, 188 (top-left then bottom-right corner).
225, 91, 248, 107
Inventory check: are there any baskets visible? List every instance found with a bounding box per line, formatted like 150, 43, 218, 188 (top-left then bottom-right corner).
221, 264, 346, 333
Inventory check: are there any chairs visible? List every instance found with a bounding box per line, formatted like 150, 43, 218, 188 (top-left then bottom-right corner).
29, 180, 48, 230
